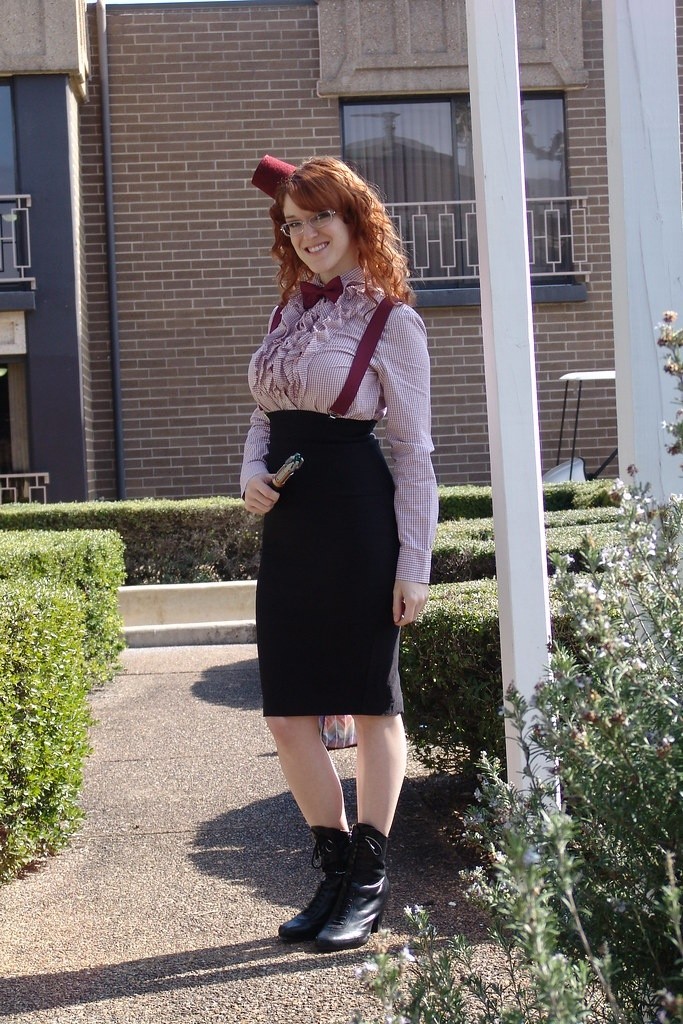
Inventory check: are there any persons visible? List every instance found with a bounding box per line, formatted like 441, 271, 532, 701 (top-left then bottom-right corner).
243, 154, 438, 950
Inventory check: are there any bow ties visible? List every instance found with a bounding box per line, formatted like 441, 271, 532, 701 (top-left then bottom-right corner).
298, 275, 344, 309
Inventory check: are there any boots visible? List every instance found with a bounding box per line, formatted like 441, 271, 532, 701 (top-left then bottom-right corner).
277, 822, 392, 949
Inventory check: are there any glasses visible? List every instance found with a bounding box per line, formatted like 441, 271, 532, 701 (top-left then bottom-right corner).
280, 209, 337, 236
